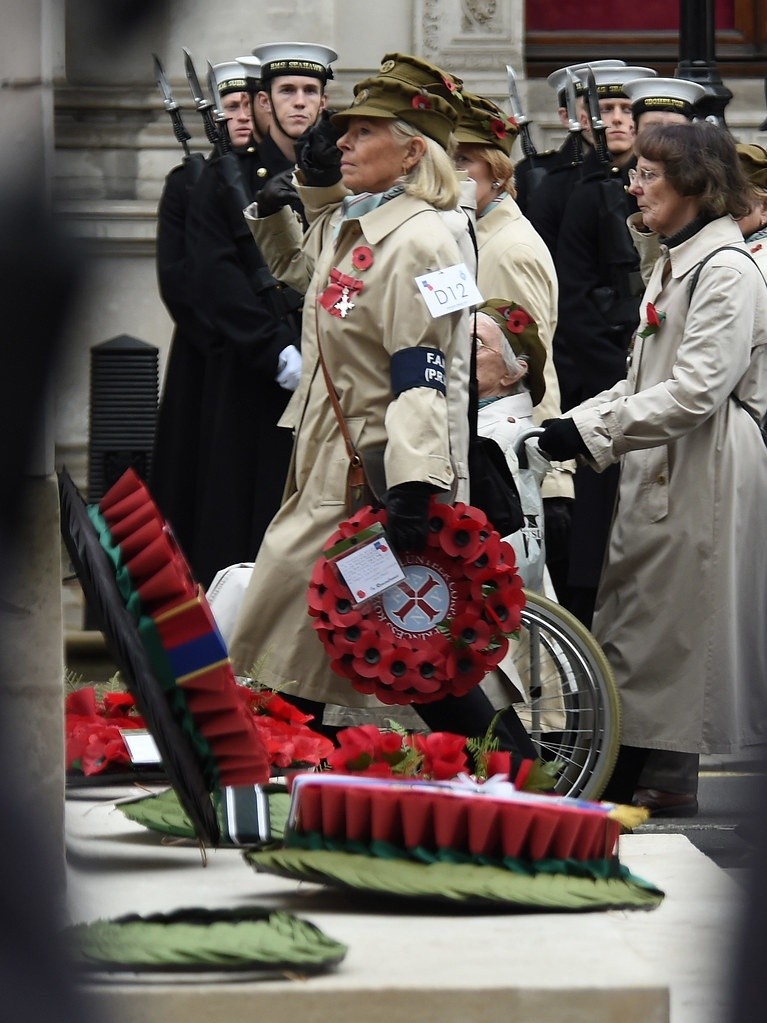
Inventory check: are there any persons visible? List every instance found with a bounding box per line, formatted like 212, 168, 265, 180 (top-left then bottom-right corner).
541, 123, 767, 816
231, 78, 485, 788
156, 42, 767, 818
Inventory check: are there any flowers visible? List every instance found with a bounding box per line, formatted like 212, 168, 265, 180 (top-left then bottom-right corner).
636, 302, 667, 340
68, 466, 669, 915
505, 309, 530, 334
481, 118, 506, 143
350, 246, 374, 274
441, 77, 466, 103
412, 96, 431, 110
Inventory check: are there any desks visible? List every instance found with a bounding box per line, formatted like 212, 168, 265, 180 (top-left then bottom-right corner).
65, 734, 748, 1023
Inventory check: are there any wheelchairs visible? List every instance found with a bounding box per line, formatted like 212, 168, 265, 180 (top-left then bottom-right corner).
416, 427, 624, 804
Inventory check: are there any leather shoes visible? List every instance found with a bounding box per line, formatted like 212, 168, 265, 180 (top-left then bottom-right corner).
632, 789, 699, 818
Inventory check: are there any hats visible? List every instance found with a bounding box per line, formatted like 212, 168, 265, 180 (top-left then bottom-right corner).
329, 78, 458, 153
621, 78, 705, 118
375, 53, 464, 134
547, 59, 625, 108
208, 62, 248, 97
734, 143, 767, 189
451, 93, 520, 157
477, 298, 547, 407
252, 43, 338, 91
235, 55, 269, 92
575, 67, 660, 103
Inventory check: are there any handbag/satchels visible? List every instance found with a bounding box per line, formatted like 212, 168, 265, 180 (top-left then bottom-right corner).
466, 434, 525, 539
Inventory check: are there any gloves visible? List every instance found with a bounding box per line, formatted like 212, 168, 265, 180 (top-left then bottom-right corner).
308, 109, 345, 167
275, 344, 304, 390
385, 484, 430, 552
257, 168, 301, 217
539, 418, 587, 462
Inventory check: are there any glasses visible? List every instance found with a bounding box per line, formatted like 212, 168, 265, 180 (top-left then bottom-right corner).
628, 168, 670, 186
